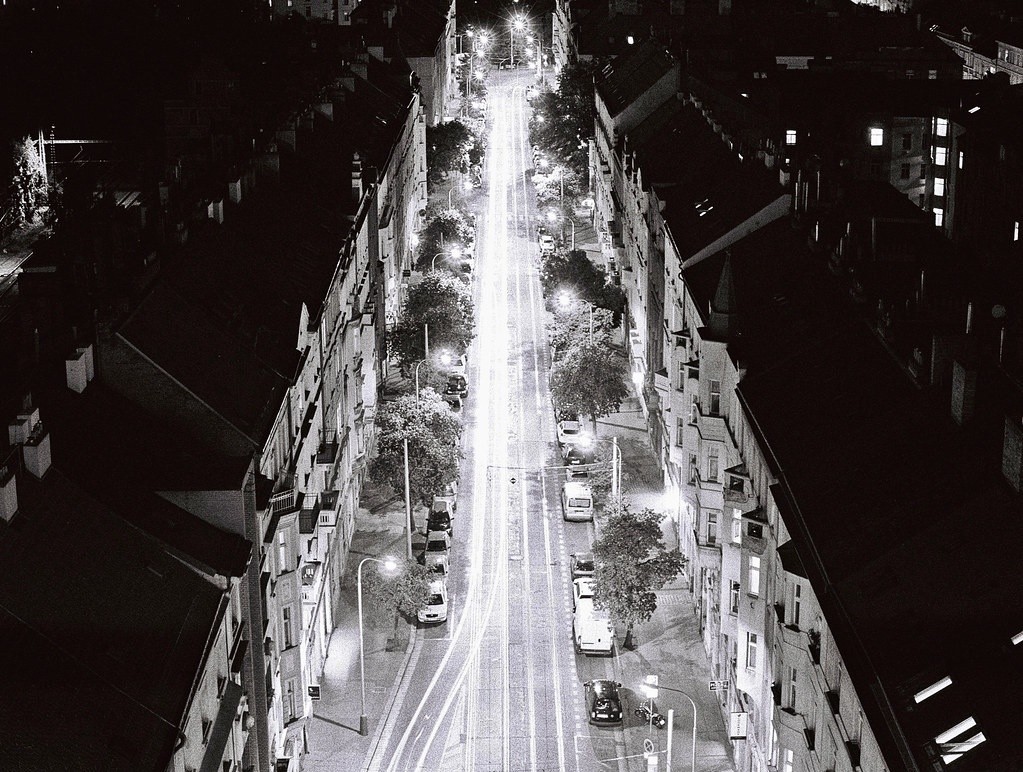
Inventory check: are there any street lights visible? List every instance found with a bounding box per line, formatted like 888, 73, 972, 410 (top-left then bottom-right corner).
558, 297, 593, 346
415, 357, 450, 412
467, 32, 488, 103
432, 252, 458, 280
357, 558, 397, 736
448, 184, 471, 212
580, 438, 622, 515
525, 36, 545, 89
549, 213, 575, 250
542, 160, 564, 204
641, 682, 697, 772
510, 16, 523, 66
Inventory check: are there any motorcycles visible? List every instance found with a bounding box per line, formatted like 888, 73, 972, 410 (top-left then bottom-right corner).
635, 702, 666, 729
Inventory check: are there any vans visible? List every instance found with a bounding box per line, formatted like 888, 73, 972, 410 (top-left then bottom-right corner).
561, 482, 593, 522
574, 601, 614, 657
426, 530, 451, 559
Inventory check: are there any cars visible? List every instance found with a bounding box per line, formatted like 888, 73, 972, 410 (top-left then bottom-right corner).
424, 556, 450, 588
564, 466, 590, 484
418, 581, 447, 624
426, 475, 457, 535
442, 351, 469, 413
570, 552, 598, 580
553, 397, 586, 465
583, 679, 624, 727
572, 577, 596, 608
531, 143, 560, 255
